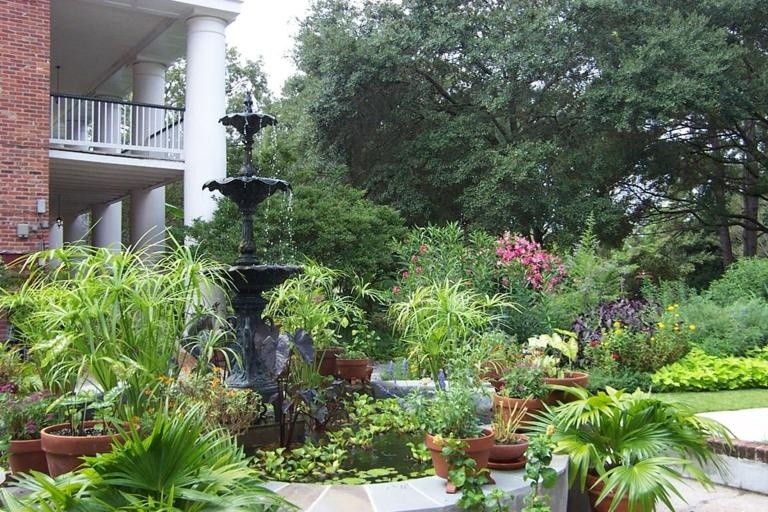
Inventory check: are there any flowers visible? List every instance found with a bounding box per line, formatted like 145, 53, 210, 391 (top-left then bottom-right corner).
0, 383, 56, 439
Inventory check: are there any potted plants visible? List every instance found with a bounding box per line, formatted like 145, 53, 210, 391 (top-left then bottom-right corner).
310, 312, 383, 384
41, 382, 142, 478
423, 326, 588, 481
519, 382, 741, 512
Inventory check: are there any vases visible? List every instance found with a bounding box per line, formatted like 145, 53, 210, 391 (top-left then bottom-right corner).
5, 439, 54, 479
